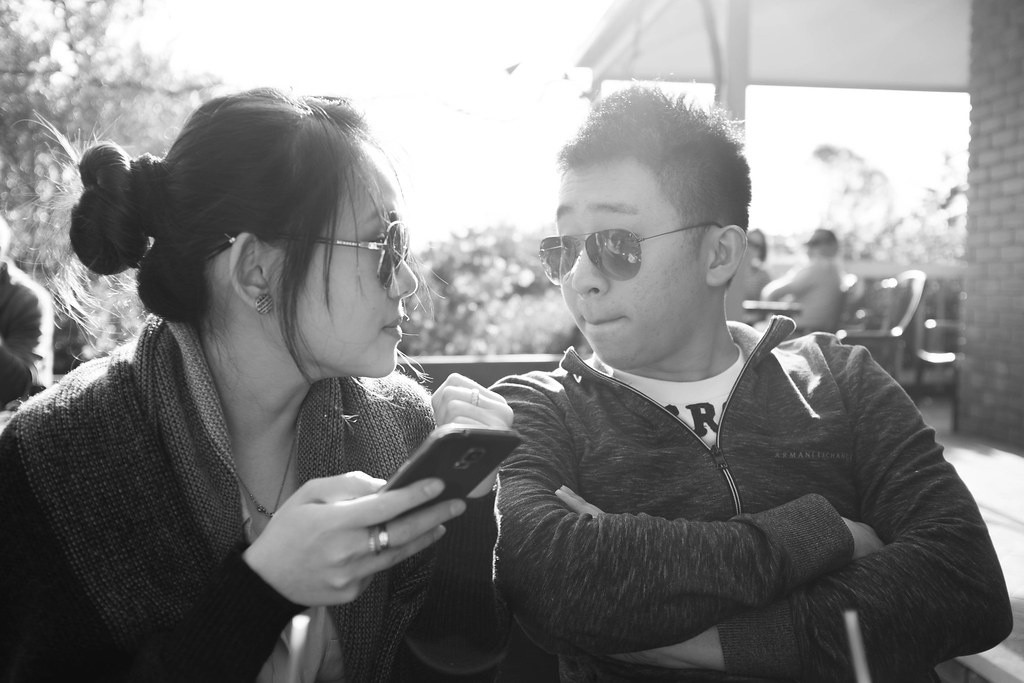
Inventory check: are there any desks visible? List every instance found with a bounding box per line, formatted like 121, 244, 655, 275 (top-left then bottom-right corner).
739, 295, 805, 330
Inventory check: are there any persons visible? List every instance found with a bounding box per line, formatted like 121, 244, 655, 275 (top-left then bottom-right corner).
1, 90, 513, 683
479, 86, 1011, 683
760, 227, 845, 342
722, 221, 773, 324
0, 216, 55, 407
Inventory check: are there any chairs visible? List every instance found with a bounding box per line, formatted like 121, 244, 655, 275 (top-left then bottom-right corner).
822, 268, 928, 400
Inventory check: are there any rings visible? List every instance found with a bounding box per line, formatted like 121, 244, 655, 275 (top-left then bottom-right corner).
379, 521, 391, 551
471, 387, 479, 407
370, 526, 379, 556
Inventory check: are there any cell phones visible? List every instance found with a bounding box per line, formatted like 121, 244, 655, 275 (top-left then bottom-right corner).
378, 424, 524, 524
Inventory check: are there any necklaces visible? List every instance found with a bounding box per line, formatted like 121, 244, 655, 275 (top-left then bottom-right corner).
233, 437, 295, 522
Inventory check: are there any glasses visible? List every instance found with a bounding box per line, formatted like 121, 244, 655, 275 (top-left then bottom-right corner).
804, 239, 827, 247
204, 220, 410, 290
746, 239, 764, 250
539, 223, 722, 288
